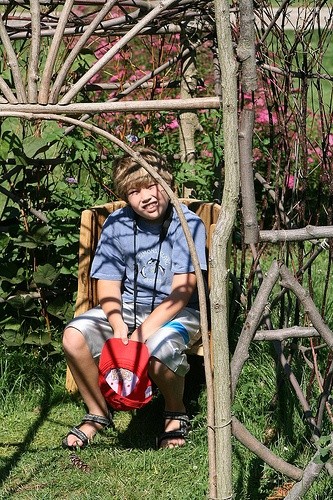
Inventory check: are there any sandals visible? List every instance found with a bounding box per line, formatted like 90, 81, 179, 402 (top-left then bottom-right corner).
62, 412, 115, 451
158, 413, 191, 451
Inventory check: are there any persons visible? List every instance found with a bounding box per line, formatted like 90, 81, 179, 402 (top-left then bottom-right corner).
60, 147, 208, 452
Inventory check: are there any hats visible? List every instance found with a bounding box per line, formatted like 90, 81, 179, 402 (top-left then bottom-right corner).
97, 338, 153, 411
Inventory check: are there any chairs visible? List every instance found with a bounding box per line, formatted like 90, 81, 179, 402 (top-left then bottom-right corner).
65, 198, 222, 397
